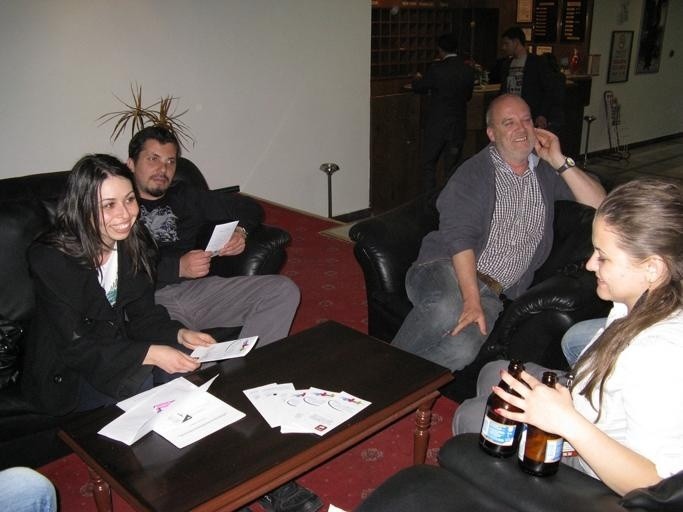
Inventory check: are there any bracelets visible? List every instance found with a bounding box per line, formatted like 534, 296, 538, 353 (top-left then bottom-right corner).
235, 226, 248, 239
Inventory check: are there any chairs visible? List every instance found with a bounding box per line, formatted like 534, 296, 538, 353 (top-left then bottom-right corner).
354, 464, 522, 512
436, 415, 683, 512
348, 200, 609, 404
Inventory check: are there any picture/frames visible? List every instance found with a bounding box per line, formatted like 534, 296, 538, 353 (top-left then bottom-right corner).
607, 29, 634, 86
634, 0, 670, 75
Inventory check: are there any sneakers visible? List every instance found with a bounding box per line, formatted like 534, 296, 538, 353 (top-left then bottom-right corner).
258, 480, 324, 511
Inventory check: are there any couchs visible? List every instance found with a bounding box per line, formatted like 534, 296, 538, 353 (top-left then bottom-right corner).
1, 158, 293, 470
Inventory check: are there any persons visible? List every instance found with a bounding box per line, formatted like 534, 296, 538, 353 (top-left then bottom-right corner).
392, 94, 608, 380
500, 27, 554, 132
410, 38, 473, 193
452, 178, 683, 498
20, 153, 218, 424
125, 127, 323, 511
560, 316, 613, 366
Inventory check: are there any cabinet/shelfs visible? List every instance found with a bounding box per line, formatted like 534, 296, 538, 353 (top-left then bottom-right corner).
370, 4, 453, 79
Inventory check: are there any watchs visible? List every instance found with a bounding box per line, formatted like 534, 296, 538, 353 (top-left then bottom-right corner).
555, 157, 576, 176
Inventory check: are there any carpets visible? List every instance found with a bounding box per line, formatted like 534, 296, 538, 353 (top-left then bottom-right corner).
319, 218, 387, 248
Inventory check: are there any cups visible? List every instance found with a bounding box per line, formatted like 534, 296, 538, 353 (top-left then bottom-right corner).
479, 71, 489, 88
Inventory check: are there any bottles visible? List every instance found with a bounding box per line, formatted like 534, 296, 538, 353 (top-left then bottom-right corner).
519, 371, 564, 478
478, 361, 525, 458
